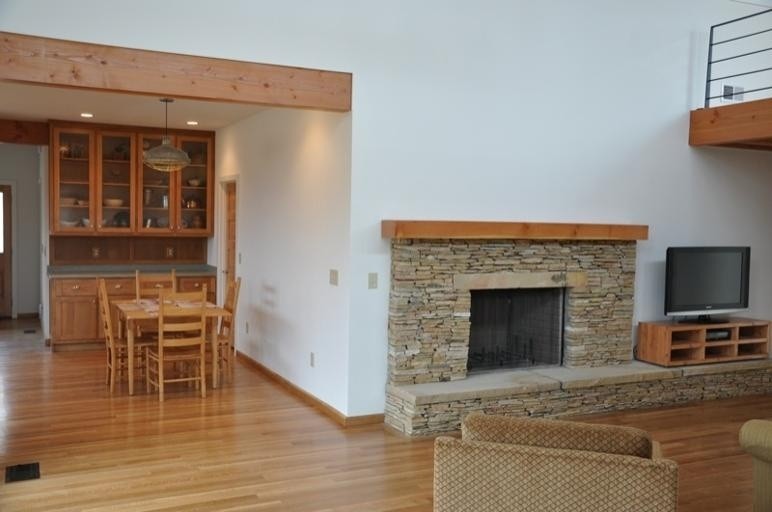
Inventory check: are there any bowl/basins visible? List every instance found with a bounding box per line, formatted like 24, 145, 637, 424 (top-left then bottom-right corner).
62, 219, 78, 226
83, 218, 105, 227
104, 199, 123, 206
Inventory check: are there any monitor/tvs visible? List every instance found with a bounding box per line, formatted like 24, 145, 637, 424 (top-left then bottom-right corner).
664, 246, 751, 324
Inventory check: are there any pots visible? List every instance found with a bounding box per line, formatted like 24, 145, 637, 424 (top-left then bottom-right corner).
186, 194, 200, 209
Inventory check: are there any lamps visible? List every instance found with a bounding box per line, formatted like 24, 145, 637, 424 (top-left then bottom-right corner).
142, 97, 192, 173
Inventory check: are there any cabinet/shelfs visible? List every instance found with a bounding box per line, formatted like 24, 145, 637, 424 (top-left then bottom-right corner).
49, 120, 216, 237
47, 274, 218, 354
635, 316, 772, 368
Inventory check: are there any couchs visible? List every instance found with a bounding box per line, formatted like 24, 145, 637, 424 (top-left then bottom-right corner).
432, 411, 680, 512
737, 418, 772, 512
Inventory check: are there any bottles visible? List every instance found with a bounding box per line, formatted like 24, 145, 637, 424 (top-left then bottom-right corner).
161, 194, 168, 208
143, 189, 152, 207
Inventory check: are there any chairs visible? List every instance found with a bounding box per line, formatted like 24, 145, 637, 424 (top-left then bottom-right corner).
95, 278, 160, 392
187, 276, 243, 390
146, 283, 211, 402
135, 267, 185, 371
